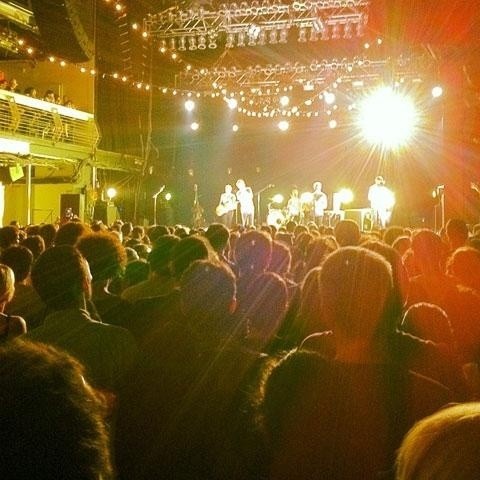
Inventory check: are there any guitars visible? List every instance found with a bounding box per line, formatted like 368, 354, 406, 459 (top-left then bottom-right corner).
215, 200, 238, 216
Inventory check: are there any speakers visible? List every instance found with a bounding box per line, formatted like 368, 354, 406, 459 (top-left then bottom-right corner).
94, 206, 117, 225
60, 193, 85, 223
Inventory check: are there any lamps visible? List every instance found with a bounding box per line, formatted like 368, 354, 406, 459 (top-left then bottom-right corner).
142, 1, 369, 54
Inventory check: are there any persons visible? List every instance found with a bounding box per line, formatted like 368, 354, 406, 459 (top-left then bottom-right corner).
218, 176, 395, 229
2, 207, 480, 480
0, 79, 78, 144
